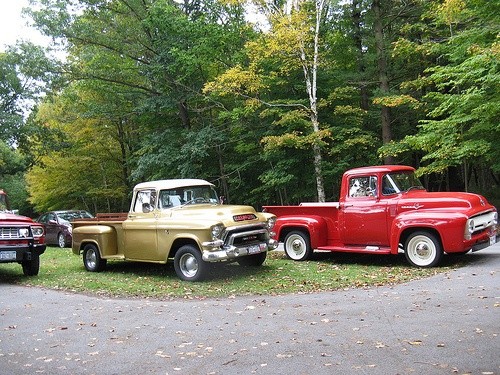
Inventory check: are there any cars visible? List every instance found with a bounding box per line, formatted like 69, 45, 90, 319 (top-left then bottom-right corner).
35, 210, 94, 248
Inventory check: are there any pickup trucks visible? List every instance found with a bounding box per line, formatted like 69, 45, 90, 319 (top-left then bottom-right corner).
69, 179, 279, 282
0, 211, 47, 277
261, 166, 498, 268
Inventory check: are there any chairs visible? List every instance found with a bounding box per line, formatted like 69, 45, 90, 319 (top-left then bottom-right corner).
168, 195, 184, 207
